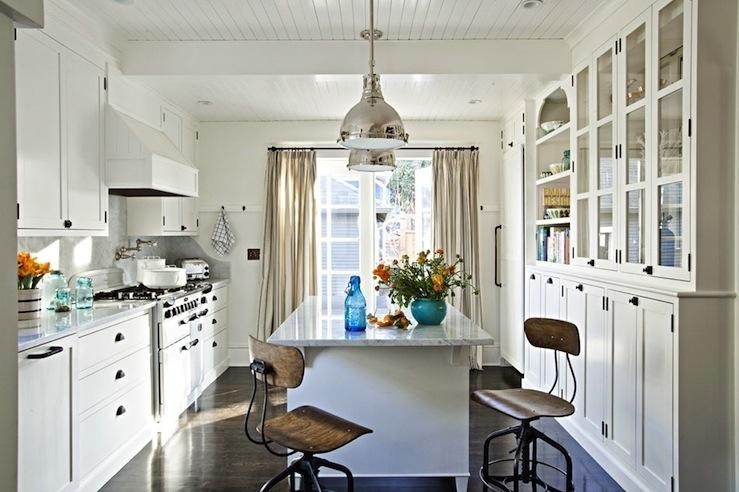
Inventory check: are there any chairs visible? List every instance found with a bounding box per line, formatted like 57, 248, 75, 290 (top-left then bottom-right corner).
243, 333, 371, 492
472, 314, 583, 492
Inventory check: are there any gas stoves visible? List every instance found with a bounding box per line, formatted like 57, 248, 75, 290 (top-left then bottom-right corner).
95, 285, 210, 301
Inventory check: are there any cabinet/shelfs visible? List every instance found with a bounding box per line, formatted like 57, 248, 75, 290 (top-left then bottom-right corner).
125, 97, 198, 237
1, 9, 111, 239
208, 283, 227, 391
0, 338, 71, 492
70, 306, 157, 491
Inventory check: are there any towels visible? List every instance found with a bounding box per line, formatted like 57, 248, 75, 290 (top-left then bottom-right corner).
210, 208, 237, 257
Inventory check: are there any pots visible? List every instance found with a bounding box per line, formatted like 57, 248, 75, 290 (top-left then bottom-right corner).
142, 265, 187, 289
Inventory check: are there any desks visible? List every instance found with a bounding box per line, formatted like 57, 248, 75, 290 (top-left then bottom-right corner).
267, 295, 493, 492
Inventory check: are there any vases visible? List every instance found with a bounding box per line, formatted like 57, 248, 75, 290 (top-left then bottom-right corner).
410, 299, 448, 327
18, 289, 44, 320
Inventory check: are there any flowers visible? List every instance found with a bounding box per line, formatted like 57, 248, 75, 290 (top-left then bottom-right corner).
15, 252, 62, 290
372, 252, 481, 301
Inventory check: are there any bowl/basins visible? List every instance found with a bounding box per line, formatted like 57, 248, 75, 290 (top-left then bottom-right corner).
549, 163, 562, 175
546, 208, 570, 218
552, 121, 569, 129
539, 120, 555, 132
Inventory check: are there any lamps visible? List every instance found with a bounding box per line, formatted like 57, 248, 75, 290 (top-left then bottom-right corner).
347, 148, 399, 172
334, 27, 409, 149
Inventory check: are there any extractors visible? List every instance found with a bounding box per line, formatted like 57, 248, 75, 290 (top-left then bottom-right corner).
107, 103, 198, 200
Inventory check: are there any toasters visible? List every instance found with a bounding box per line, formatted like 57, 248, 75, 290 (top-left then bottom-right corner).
182, 260, 210, 280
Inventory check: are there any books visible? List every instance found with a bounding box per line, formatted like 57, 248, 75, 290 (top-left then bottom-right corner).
536, 225, 569, 265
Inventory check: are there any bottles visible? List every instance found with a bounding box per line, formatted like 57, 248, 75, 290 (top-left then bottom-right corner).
659, 222, 675, 265
45, 270, 67, 310
75, 277, 95, 309
344, 275, 367, 331
344, 332, 367, 340
55, 288, 72, 312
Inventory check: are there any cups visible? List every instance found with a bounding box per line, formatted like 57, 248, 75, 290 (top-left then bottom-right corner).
562, 149, 570, 171
